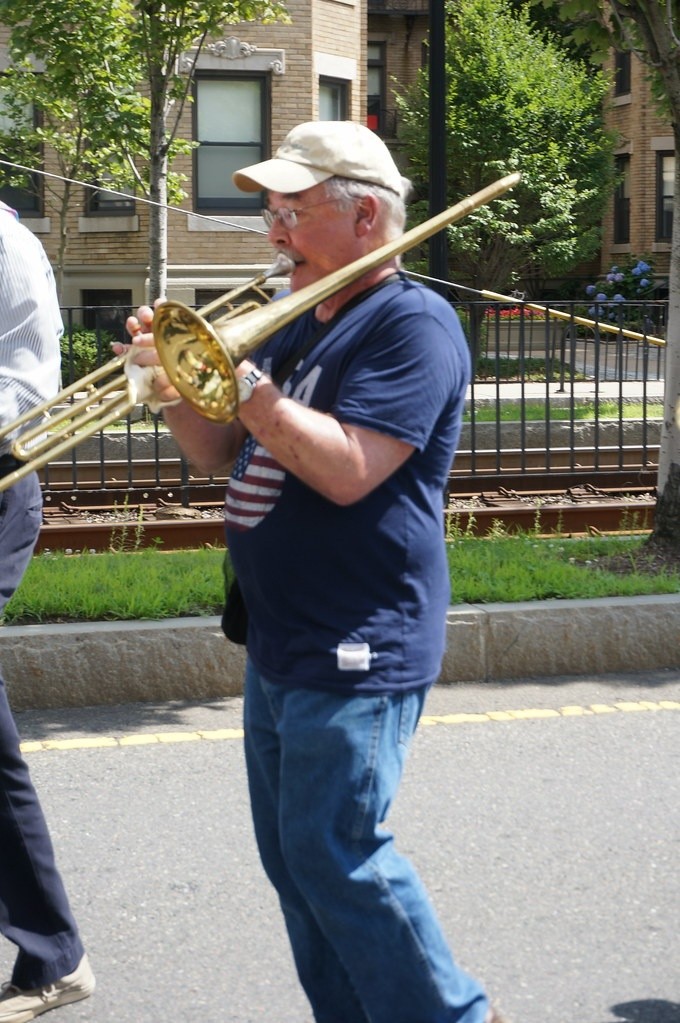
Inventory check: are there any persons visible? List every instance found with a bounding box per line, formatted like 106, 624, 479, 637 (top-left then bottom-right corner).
0, 198, 96, 1023
110, 121, 507, 1023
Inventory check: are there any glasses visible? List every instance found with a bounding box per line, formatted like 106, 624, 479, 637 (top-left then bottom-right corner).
262, 198, 341, 228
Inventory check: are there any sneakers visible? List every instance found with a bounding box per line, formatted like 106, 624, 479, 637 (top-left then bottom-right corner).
1, 953, 95, 1022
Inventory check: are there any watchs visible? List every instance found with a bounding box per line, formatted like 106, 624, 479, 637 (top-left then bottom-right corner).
236, 367, 262, 406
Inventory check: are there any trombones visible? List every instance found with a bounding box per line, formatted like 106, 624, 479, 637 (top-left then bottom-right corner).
0, 173, 521, 491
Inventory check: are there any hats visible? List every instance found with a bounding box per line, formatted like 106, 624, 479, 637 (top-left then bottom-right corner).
233, 119, 404, 196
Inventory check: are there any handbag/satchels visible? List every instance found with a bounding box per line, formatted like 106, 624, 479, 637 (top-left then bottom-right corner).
221, 548, 249, 644
